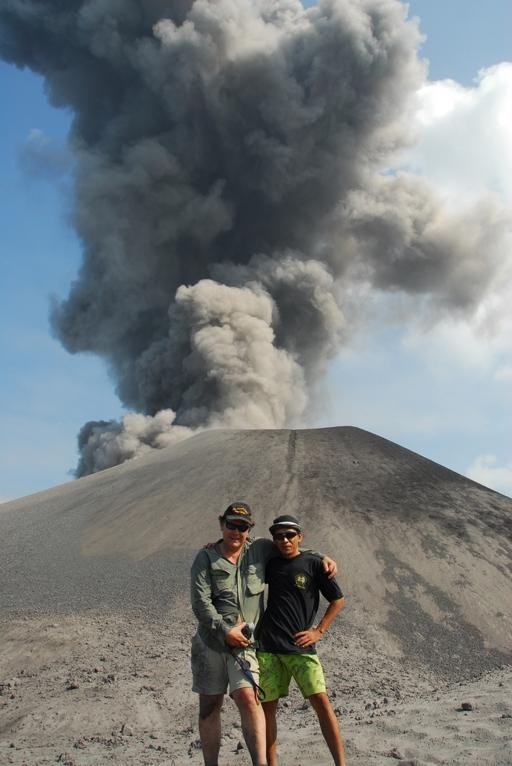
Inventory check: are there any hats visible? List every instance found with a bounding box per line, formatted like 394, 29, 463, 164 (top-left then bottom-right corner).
224, 502, 253, 526
269, 515, 302, 536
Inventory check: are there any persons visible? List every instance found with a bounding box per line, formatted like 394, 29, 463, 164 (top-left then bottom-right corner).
190, 502, 338, 766
202, 514, 346, 766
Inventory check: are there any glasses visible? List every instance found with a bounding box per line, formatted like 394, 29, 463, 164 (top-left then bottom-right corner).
225, 520, 248, 532
274, 532, 297, 540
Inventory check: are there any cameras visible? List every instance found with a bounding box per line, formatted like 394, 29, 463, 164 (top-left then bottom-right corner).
241, 624, 252, 640
